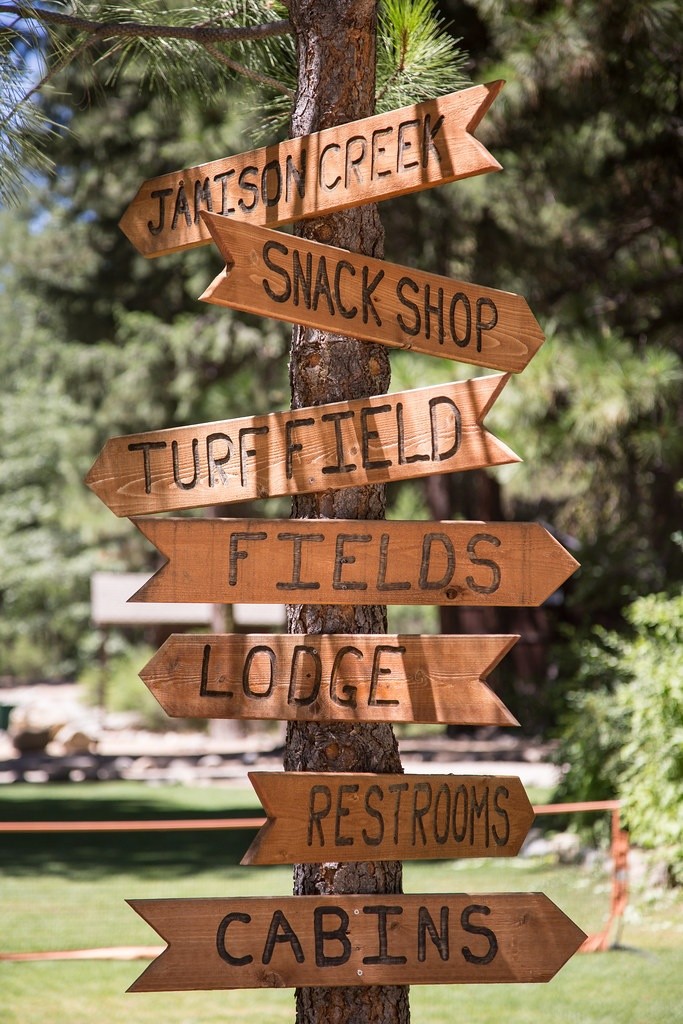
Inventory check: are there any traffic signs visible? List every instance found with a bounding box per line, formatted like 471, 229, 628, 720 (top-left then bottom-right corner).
239, 771, 536, 865
126, 516, 581, 607
138, 634, 521, 729
117, 78, 508, 259
198, 210, 546, 373
85, 374, 524, 518
124, 892, 589, 993
